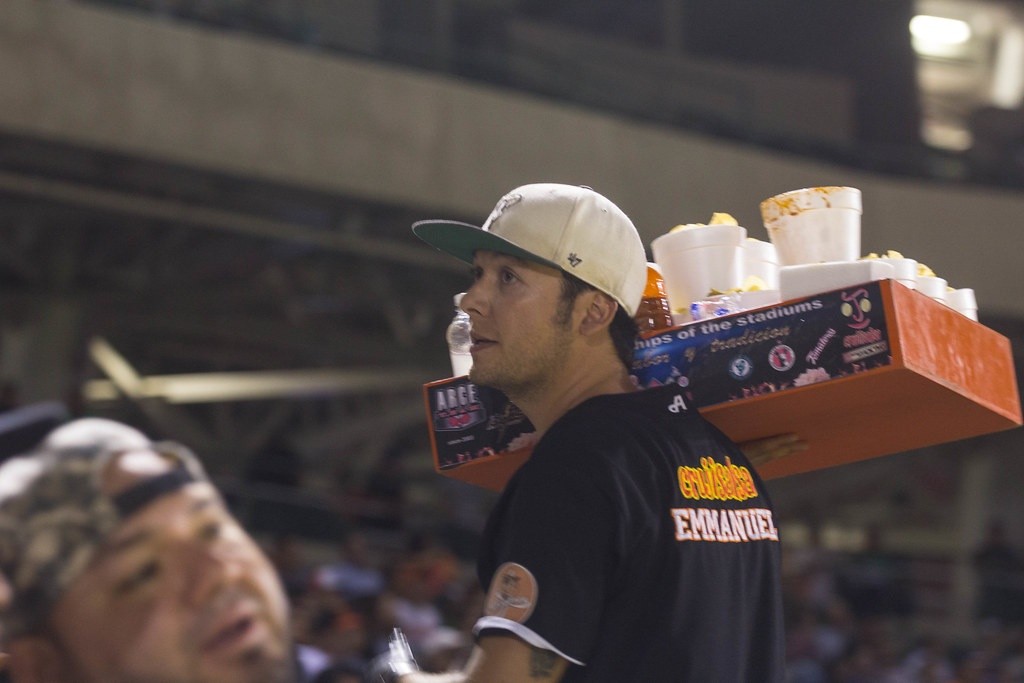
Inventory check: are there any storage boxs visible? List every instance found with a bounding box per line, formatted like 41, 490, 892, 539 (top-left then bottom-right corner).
423, 277, 1023, 487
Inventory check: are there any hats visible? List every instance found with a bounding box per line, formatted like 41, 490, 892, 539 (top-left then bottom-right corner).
412, 184, 649, 317
0, 418, 212, 622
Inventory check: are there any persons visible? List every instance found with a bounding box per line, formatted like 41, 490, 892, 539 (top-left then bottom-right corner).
273, 529, 472, 683
1, 416, 297, 682
785, 518, 1024, 682
367, 183, 785, 683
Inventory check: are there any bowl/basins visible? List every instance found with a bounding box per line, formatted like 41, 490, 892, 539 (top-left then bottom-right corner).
757, 183, 862, 266
865, 249, 979, 323
652, 225, 748, 311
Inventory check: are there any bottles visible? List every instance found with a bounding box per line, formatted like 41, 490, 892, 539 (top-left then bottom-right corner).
444, 291, 476, 376
624, 265, 674, 334
